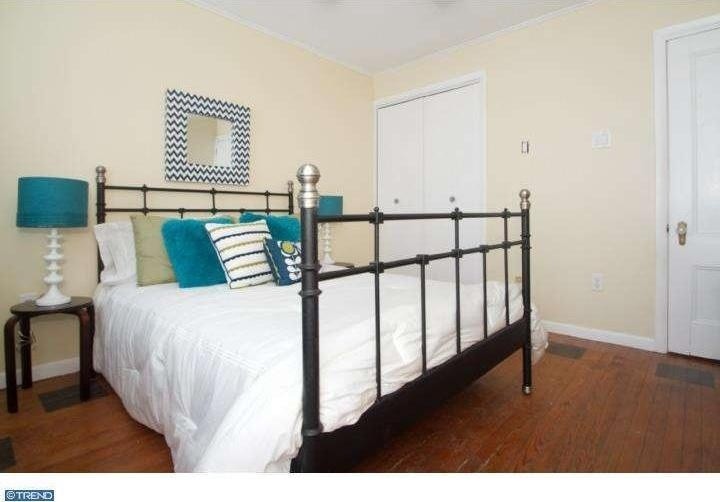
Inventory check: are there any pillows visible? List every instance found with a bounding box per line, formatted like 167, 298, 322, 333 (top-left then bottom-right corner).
94, 209, 305, 291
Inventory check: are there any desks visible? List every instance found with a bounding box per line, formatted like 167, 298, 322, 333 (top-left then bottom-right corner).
4, 297, 97, 414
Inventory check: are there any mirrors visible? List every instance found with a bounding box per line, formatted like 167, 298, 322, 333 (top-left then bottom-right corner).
164, 88, 254, 190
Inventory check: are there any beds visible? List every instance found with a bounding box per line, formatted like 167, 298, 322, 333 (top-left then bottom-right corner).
89, 164, 535, 474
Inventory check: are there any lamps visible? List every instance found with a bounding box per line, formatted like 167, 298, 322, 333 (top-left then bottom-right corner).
15, 175, 90, 307
316, 191, 345, 272
